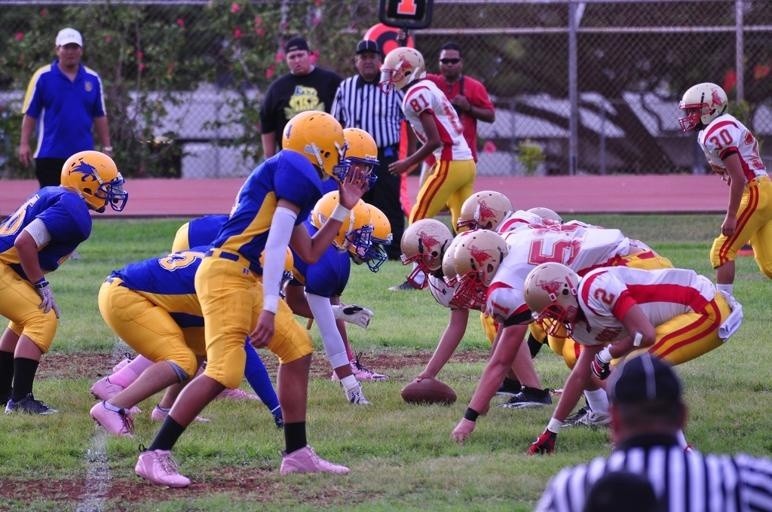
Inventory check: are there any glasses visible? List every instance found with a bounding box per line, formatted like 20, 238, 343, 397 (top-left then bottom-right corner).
440, 58, 462, 64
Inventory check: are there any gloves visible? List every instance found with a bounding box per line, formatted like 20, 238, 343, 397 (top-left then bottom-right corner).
591, 354, 612, 380
526, 430, 557, 455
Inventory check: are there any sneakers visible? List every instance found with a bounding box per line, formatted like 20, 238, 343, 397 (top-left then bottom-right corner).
331, 352, 389, 381
4, 393, 57, 415
278, 445, 349, 475
89, 358, 261, 487
494, 375, 610, 428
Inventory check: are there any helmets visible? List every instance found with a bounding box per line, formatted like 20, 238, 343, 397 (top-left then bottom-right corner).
379, 47, 427, 92
678, 82, 729, 133
60, 149, 128, 214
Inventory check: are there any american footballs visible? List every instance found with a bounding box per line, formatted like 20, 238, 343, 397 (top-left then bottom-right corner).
402, 376, 458, 405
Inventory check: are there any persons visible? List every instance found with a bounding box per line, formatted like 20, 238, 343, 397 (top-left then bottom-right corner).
678, 79, 772, 294
531, 353, 772, 512
257, 36, 496, 290
0, 149, 128, 417
15, 27, 114, 261
88, 109, 745, 491
584, 471, 663, 512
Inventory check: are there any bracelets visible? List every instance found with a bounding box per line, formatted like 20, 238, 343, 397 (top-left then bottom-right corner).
103, 143, 112, 151
34, 281, 50, 288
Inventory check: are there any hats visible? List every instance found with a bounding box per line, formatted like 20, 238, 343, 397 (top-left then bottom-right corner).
607, 353, 682, 403
355, 40, 381, 53
55, 27, 83, 48
285, 37, 309, 52
